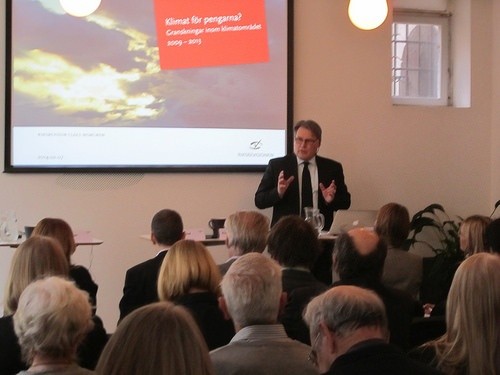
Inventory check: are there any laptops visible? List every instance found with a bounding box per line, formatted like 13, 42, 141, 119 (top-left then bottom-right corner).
321, 210, 378, 235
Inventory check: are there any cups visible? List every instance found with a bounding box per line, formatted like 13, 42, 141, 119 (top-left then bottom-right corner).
304, 207, 324, 236
208, 219, 225, 237
25, 226, 35, 239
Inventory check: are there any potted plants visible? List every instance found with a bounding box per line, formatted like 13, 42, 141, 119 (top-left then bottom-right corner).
405, 197, 500, 312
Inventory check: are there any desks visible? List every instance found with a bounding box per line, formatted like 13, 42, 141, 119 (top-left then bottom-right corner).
140, 230, 340, 283
0, 230, 104, 248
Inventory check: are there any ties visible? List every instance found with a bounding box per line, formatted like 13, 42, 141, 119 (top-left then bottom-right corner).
300, 161, 313, 220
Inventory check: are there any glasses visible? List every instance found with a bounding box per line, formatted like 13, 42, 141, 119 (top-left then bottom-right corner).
294, 135, 318, 146
306, 326, 336, 367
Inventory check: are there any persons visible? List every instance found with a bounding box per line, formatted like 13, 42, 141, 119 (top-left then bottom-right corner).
267, 215, 330, 347
92, 302, 216, 375
13, 275, 97, 375
116, 209, 187, 328
329, 226, 424, 351
0, 236, 111, 375
217, 209, 270, 279
156, 238, 238, 352
421, 215, 492, 335
302, 285, 448, 375
31, 218, 99, 314
407, 253, 500, 375
373, 203, 424, 299
209, 251, 320, 375
253, 119, 352, 287
483, 220, 500, 253
